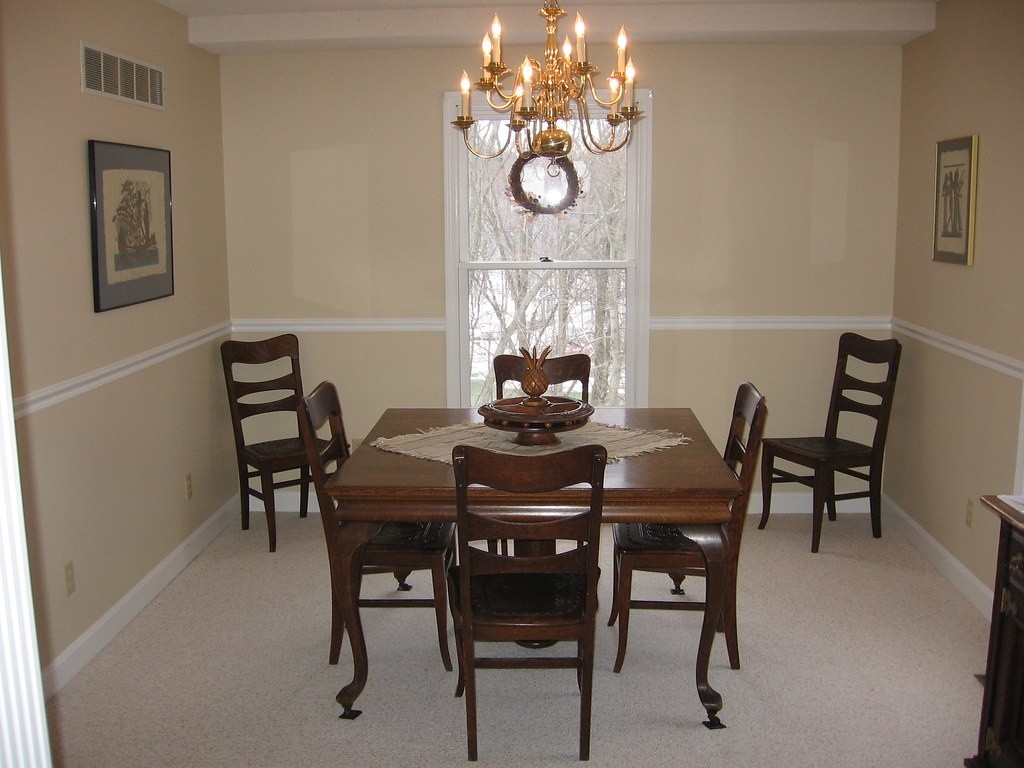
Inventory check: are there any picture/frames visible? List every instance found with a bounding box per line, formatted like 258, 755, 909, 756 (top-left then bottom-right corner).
88, 139, 175, 313
931, 134, 979, 266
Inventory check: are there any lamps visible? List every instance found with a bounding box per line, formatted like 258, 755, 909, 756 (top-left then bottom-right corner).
449, 0, 644, 178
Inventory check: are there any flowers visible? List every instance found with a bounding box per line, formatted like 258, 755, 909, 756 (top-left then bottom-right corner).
505, 150, 586, 223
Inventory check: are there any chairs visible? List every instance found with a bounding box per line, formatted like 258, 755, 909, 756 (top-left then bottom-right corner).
447, 444, 607, 761
488, 354, 591, 556
608, 382, 768, 673
757, 332, 902, 553
221, 332, 350, 553
298, 380, 457, 672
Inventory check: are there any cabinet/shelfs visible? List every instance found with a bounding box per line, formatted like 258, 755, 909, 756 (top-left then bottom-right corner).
963, 494, 1024, 768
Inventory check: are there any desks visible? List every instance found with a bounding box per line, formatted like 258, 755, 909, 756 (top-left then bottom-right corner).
324, 408, 744, 730
